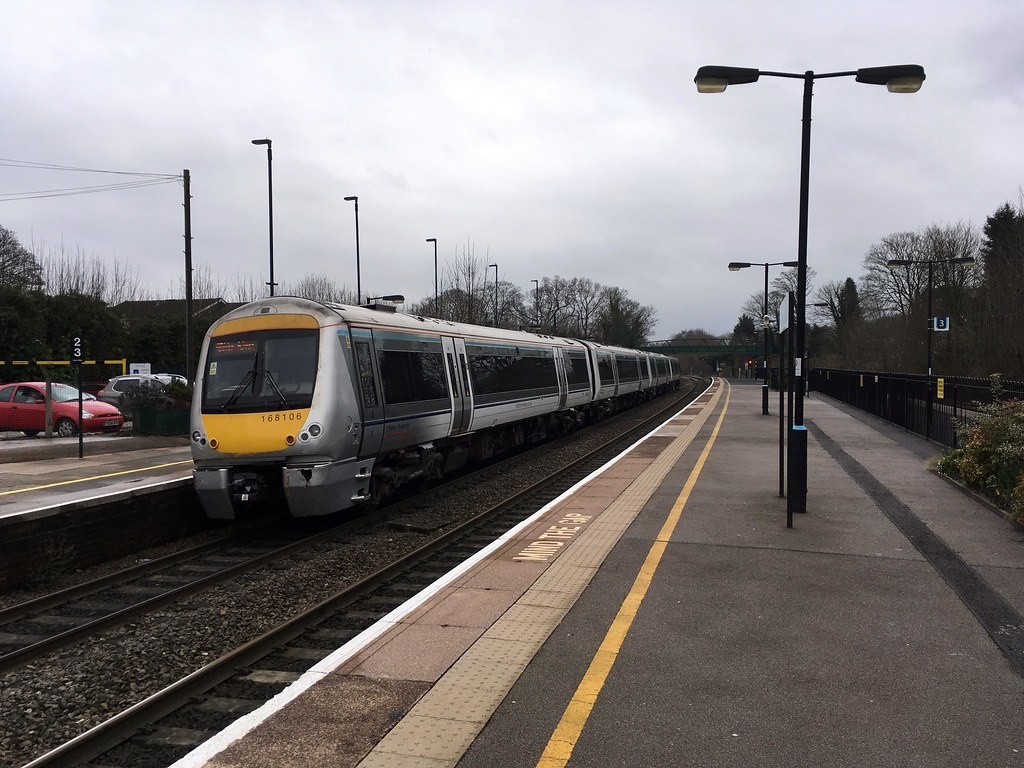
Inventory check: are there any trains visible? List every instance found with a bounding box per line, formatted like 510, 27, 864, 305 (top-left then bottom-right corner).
189, 296, 681, 527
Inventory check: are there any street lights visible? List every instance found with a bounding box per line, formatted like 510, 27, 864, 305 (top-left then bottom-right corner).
728, 261, 808, 415
886, 257, 975, 423
531, 280, 538, 333
694, 65, 927, 512
252, 139, 274, 297
367, 295, 404, 305
426, 239, 437, 318
489, 265, 498, 327
345, 196, 360, 305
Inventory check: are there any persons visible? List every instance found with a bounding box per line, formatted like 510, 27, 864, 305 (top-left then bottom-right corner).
260, 362, 280, 397
25, 391, 43, 403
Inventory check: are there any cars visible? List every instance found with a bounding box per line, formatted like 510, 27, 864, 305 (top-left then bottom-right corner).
82, 373, 187, 407
0, 382, 124, 438
